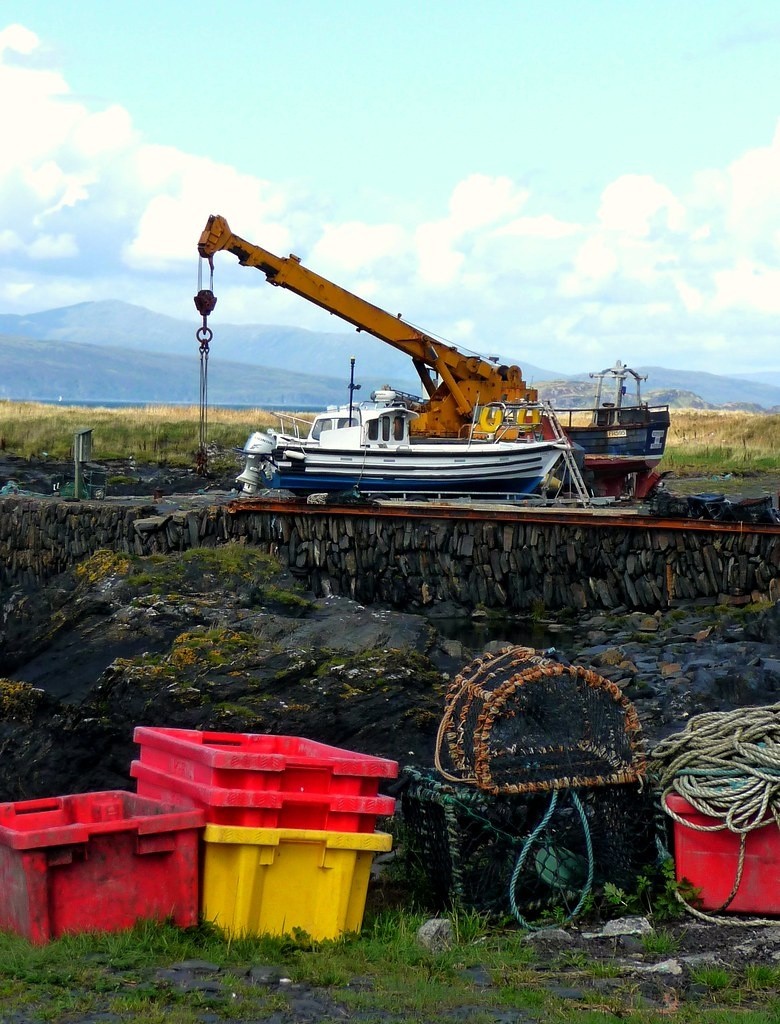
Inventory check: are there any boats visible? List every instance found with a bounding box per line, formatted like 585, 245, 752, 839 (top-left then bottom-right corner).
522, 360, 672, 496
224, 354, 575, 502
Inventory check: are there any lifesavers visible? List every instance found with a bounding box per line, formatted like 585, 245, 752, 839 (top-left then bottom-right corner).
517, 408, 540, 432
480, 407, 504, 433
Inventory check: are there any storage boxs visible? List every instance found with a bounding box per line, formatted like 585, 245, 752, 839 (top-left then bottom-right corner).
663, 794, 780, 911
0, 725, 399, 947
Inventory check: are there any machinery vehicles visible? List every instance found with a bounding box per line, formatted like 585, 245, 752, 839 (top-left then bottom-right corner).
191, 210, 545, 441
